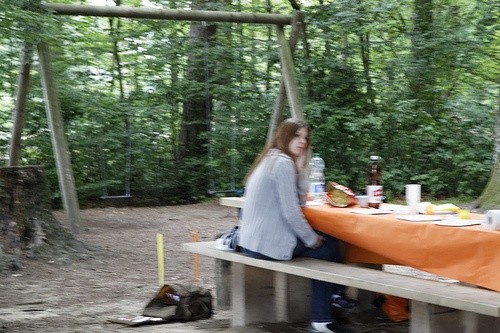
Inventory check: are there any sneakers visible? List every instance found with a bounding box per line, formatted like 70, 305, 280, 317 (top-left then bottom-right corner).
309, 321, 355, 333
330, 292, 359, 309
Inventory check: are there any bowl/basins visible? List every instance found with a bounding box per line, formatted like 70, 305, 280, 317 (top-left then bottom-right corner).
356, 195, 368, 207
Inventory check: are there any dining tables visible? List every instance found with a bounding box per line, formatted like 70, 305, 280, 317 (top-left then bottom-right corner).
220, 197, 500, 291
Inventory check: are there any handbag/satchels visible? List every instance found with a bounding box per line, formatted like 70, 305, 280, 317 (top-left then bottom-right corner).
371, 292, 410, 321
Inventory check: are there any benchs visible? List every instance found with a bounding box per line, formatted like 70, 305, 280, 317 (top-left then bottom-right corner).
182, 241, 500, 333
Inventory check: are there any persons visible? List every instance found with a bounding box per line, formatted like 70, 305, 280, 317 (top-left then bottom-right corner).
236, 117, 360, 333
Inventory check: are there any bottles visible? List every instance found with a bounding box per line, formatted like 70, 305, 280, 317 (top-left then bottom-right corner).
310, 153, 326, 200
366, 156, 382, 209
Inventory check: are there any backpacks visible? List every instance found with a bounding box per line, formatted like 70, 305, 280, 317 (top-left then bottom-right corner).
107, 282, 213, 327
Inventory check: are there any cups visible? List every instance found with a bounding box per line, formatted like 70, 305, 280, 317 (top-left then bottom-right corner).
405, 184, 431, 215
486, 209, 500, 230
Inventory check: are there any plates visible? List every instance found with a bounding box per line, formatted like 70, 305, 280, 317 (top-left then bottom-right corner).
434, 211, 457, 215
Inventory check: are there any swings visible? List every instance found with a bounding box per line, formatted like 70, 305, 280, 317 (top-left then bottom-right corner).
206, 21, 242, 198
99, 31, 132, 199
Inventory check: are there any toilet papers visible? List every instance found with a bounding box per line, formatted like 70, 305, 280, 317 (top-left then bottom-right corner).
483, 210, 500, 230
